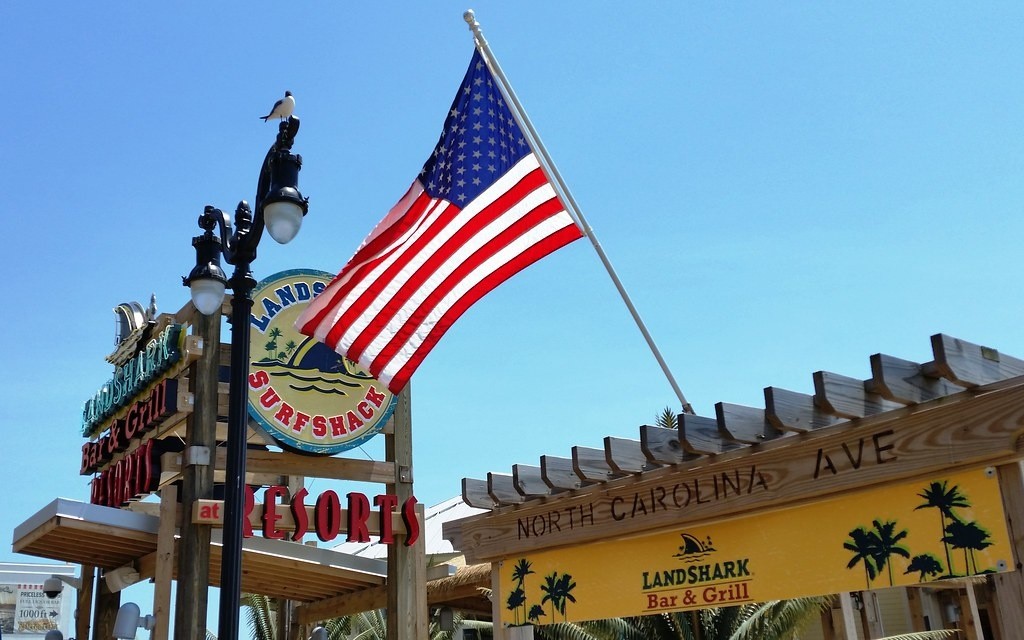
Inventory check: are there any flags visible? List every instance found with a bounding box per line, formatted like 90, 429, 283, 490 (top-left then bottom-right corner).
292, 44, 587, 396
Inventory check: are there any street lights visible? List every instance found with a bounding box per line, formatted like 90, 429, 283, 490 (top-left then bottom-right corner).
183, 117, 307, 640
